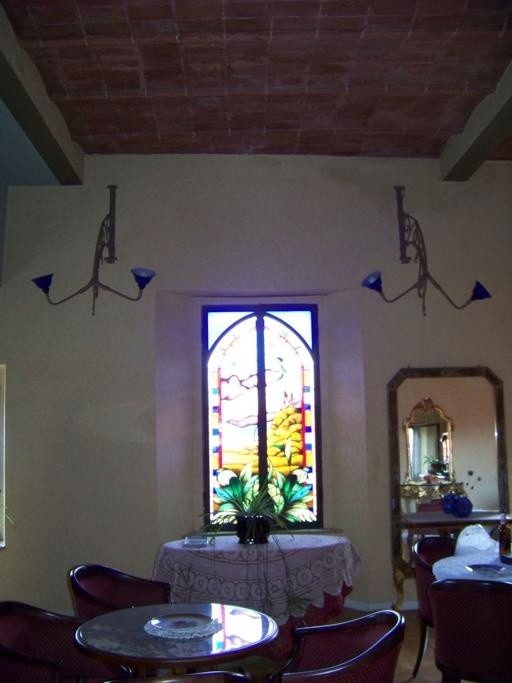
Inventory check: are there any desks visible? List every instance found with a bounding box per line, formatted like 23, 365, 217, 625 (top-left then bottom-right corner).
72, 601, 279, 670
392, 511, 512, 612
400, 483, 465, 513
152, 533, 360, 612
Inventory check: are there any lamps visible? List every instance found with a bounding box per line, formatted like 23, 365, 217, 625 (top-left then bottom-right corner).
32, 186, 156, 315
361, 187, 489, 316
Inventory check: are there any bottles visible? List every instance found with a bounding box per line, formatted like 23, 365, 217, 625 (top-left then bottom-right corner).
440, 490, 473, 518
498, 515, 512, 555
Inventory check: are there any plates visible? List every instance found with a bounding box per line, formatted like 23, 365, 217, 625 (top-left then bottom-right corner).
184, 535, 208, 547
465, 564, 506, 576
143, 612, 222, 640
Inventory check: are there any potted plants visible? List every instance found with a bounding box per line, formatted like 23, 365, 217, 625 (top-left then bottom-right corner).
424, 454, 449, 470
196, 478, 310, 544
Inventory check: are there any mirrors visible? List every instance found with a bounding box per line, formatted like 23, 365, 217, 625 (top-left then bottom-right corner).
388, 367, 512, 610
402, 397, 454, 485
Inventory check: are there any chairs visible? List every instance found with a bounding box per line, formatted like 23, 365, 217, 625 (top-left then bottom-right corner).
0, 600, 135, 680
282, 609, 406, 682
147, 670, 252, 683
65, 563, 170, 615
407, 537, 457, 683
428, 579, 511, 682
